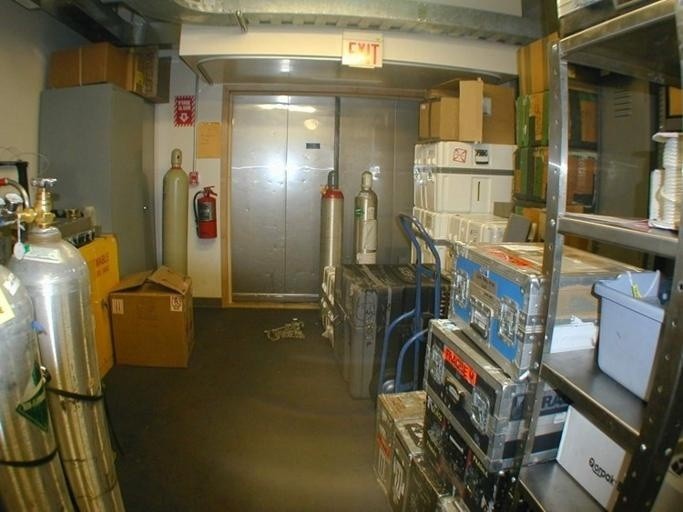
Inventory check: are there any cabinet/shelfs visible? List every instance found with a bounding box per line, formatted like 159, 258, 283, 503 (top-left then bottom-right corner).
506, 0, 683, 512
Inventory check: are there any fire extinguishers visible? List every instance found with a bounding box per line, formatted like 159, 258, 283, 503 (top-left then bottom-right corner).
193, 186, 217, 239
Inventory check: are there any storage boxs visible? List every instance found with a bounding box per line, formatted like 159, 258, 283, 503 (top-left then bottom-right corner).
46, 41, 170, 102
413, 34, 597, 275
555, 406, 625, 511
591, 272, 667, 401
108, 267, 194, 369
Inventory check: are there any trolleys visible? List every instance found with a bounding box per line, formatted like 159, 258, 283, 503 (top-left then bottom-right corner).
375, 213, 443, 402
